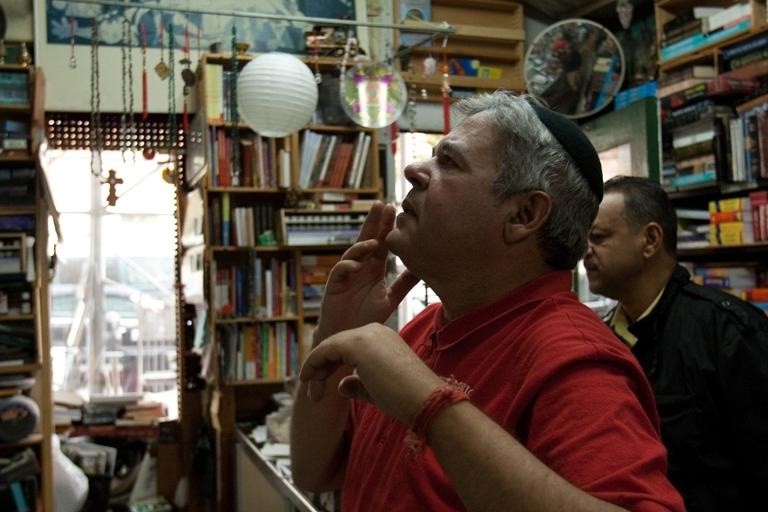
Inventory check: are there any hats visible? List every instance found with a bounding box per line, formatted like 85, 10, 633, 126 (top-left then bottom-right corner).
527, 101, 604, 203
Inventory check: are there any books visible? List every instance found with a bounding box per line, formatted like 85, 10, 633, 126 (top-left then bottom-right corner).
1, 65, 47, 512
206, 64, 382, 382
655, 1, 766, 314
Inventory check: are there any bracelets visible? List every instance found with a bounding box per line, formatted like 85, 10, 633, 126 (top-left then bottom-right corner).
410, 372, 473, 442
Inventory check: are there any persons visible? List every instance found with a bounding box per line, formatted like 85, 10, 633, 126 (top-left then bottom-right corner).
586, 172, 766, 512
285, 86, 693, 510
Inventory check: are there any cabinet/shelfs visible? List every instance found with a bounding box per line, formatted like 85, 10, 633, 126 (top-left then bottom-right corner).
651, 2, 768, 261
0, 62, 53, 512
172, 49, 393, 395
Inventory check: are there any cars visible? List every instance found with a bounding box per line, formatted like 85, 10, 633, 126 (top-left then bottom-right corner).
48, 257, 175, 392
